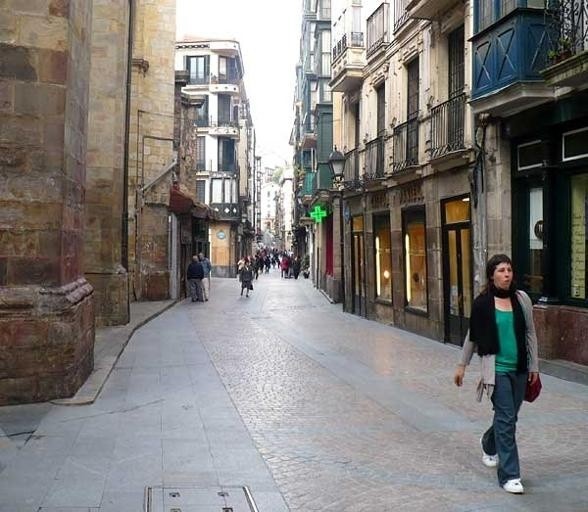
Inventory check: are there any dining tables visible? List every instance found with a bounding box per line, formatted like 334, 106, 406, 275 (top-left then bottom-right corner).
546, 37, 573, 64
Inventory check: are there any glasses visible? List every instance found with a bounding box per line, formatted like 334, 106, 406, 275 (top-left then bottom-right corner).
328, 144, 366, 195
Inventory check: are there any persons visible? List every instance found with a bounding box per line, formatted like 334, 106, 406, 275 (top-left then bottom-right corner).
455, 254, 540, 496
237, 247, 305, 280
199, 252, 212, 302
187, 255, 205, 301
240, 259, 252, 297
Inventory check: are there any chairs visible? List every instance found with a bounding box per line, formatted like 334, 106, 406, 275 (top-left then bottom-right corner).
192, 298, 208, 302
241, 293, 248, 297
502, 477, 524, 495
480, 436, 499, 468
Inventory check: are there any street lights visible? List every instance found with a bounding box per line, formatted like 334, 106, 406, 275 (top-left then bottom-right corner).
523, 373, 543, 403
250, 285, 253, 290
